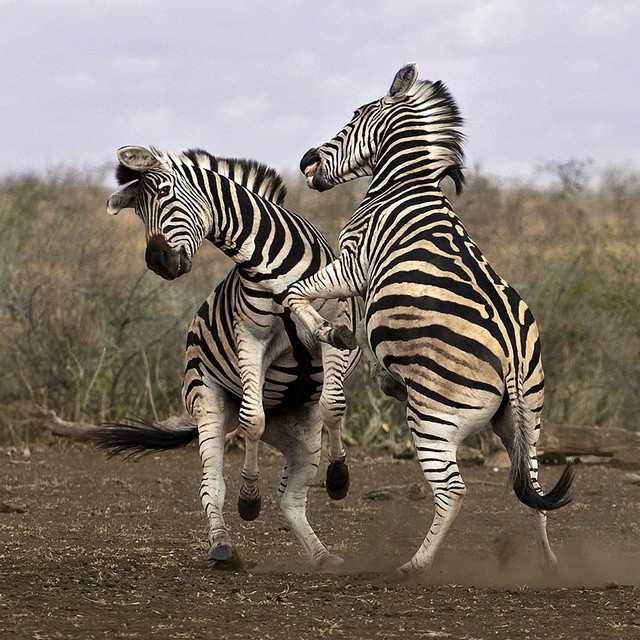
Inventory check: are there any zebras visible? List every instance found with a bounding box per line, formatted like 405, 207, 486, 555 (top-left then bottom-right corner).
288, 62, 576, 582
74, 145, 365, 571
